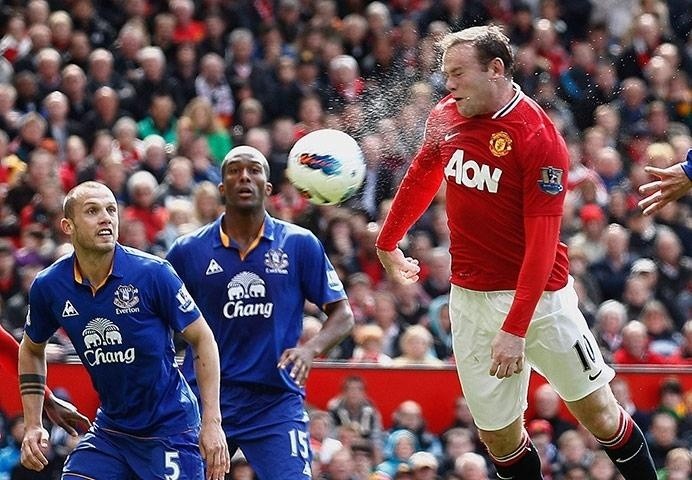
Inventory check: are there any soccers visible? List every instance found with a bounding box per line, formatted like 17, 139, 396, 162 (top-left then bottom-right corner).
285, 128, 367, 206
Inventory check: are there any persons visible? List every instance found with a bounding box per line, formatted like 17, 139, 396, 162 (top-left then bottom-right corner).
0, 1, 691, 480
